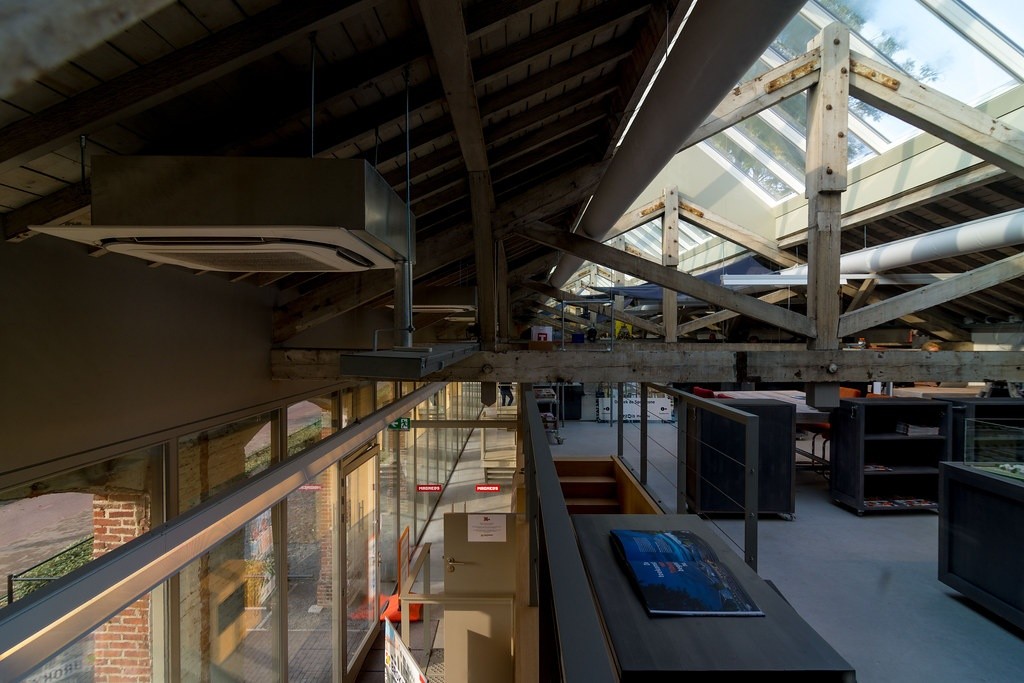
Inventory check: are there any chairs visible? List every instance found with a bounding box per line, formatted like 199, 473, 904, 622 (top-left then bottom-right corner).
813, 386, 859, 479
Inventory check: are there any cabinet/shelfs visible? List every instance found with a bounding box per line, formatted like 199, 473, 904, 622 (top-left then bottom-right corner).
829, 398, 954, 516
683, 398, 797, 518
931, 397, 1024, 464
938, 461, 1024, 628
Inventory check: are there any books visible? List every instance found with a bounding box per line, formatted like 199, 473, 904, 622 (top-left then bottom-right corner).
896, 418, 940, 437
610, 529, 764, 619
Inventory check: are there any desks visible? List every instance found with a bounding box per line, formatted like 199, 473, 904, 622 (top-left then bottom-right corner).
576, 513, 859, 683
711, 389, 832, 477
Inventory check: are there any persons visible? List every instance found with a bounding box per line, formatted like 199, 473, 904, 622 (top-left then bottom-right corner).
587, 328, 597, 343
498, 382, 514, 406
